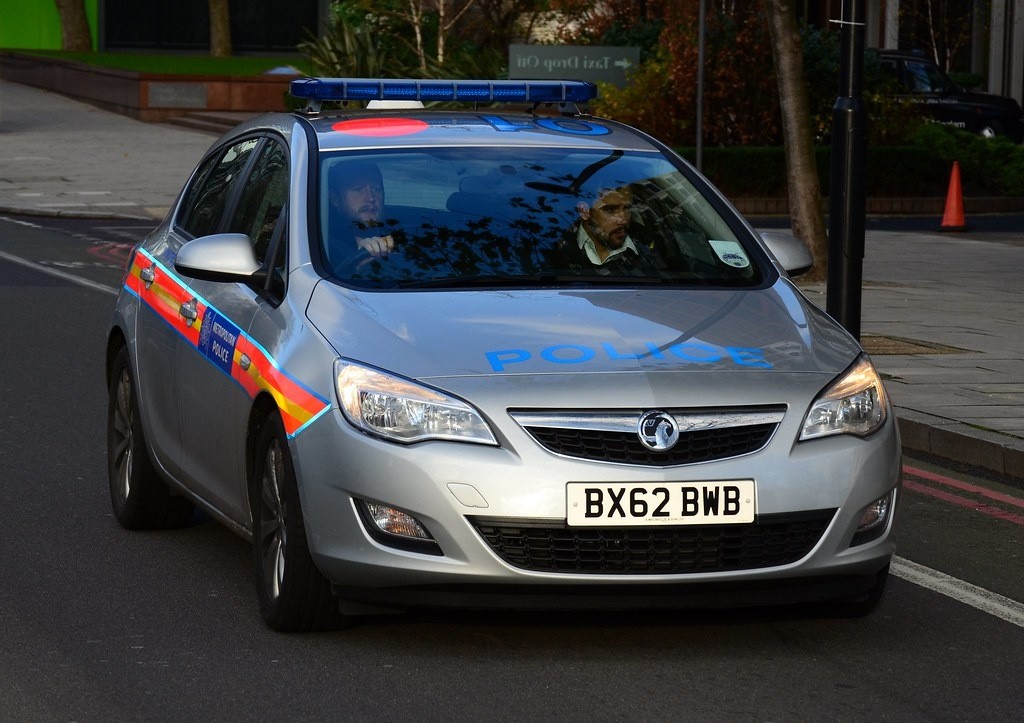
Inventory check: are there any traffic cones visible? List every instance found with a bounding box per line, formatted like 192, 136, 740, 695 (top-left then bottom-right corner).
934, 161, 971, 233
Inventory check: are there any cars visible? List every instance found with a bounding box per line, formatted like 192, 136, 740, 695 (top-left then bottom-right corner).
874, 42, 1024, 145
101, 79, 906, 630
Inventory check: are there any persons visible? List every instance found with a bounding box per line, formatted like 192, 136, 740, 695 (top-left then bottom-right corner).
524, 169, 675, 274
329, 159, 408, 277
